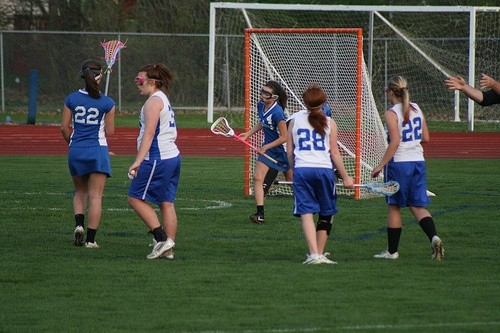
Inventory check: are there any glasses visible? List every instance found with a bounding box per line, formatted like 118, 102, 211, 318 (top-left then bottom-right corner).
135, 76, 145, 86
260, 89, 272, 99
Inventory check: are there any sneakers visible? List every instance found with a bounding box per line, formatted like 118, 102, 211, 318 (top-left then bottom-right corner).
374, 251, 399, 260
302, 254, 338, 265
249, 214, 265, 224
146, 237, 175, 259
431, 235, 445, 261
85, 241, 100, 248
73, 225, 85, 248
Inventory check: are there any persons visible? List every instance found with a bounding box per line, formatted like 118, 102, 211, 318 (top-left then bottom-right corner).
126, 63, 182, 259
233, 81, 299, 224
445, 73, 500, 106
371, 75, 445, 262
285, 85, 356, 265
61, 59, 115, 248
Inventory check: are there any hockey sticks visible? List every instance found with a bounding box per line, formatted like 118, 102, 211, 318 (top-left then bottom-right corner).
128, 170, 135, 180
273, 180, 400, 197
210, 117, 283, 167
101, 40, 125, 96
